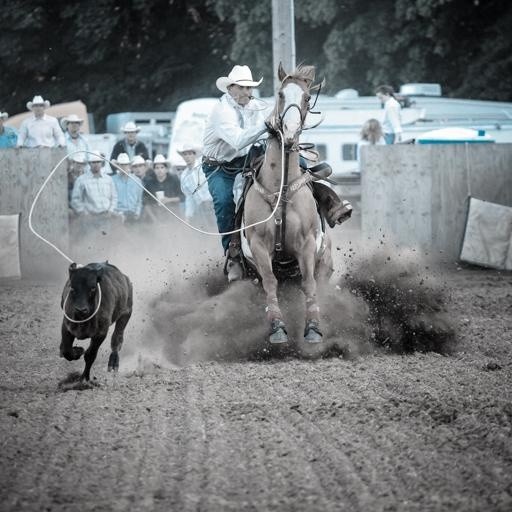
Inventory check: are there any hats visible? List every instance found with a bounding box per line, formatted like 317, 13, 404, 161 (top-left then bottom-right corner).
215, 63, 263, 93
60, 114, 84, 131
71, 151, 106, 166
177, 142, 201, 158
116, 152, 148, 168
149, 154, 171, 172
0, 111, 8, 120
26, 94, 50, 113
120, 121, 141, 134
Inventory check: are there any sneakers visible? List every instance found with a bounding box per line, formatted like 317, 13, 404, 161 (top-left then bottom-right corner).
226, 258, 243, 283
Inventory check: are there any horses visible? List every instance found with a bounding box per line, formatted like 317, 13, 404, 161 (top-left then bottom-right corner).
243, 60, 326, 346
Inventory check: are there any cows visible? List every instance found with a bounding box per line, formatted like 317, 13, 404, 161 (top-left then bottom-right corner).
59, 260, 133, 386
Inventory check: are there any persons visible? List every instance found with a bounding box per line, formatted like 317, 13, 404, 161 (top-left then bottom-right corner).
356, 120, 386, 173
204, 65, 332, 285
0, 95, 211, 221
376, 86, 403, 145
